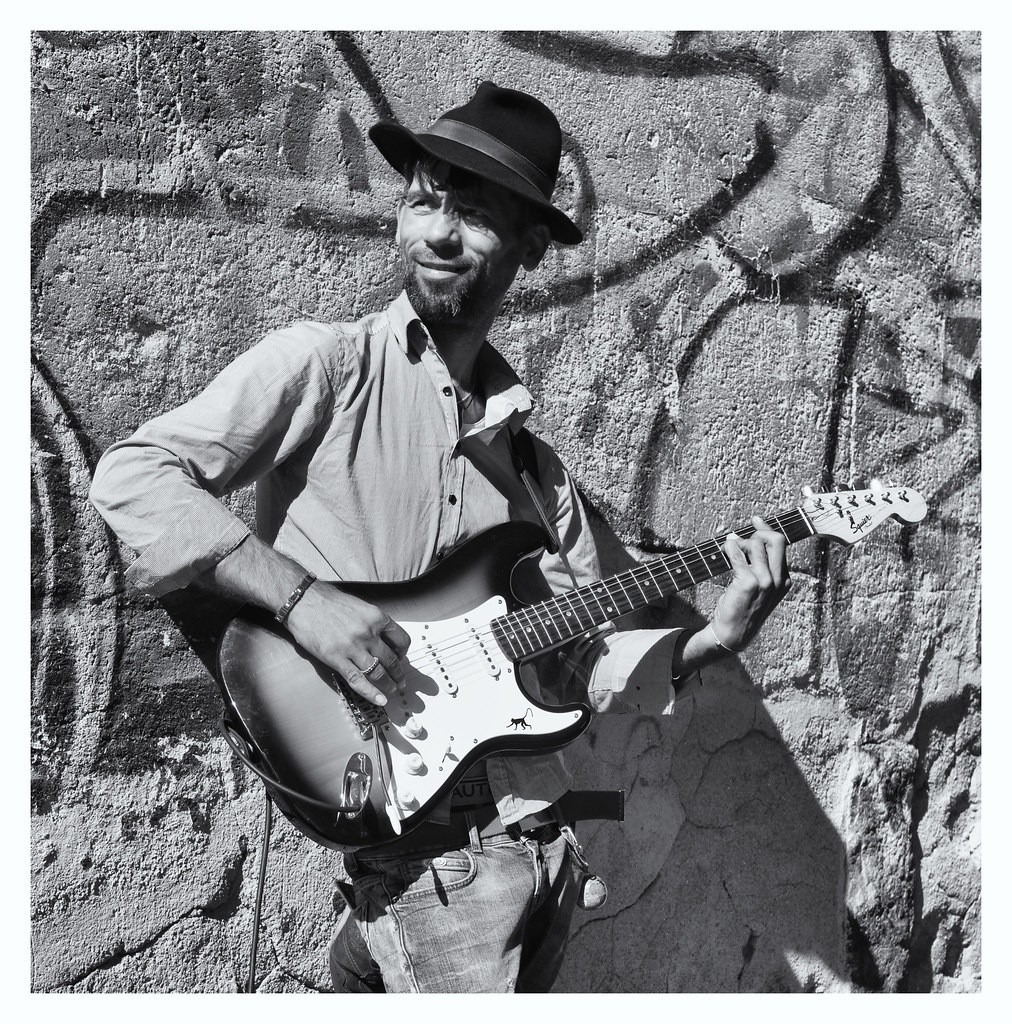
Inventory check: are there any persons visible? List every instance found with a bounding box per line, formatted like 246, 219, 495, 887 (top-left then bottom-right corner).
88, 81, 792, 993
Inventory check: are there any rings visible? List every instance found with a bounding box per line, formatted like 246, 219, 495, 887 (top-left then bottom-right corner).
360, 657, 380, 674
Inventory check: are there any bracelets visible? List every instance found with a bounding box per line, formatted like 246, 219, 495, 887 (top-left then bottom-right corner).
273, 571, 318, 624
710, 623, 744, 653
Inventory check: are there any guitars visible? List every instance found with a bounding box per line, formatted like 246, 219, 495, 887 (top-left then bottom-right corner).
218, 478, 929, 854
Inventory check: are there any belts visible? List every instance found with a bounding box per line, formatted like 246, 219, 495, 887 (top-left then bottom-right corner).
352, 788, 627, 861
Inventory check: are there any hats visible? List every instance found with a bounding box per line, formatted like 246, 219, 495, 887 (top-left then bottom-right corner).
368, 81, 583, 245
